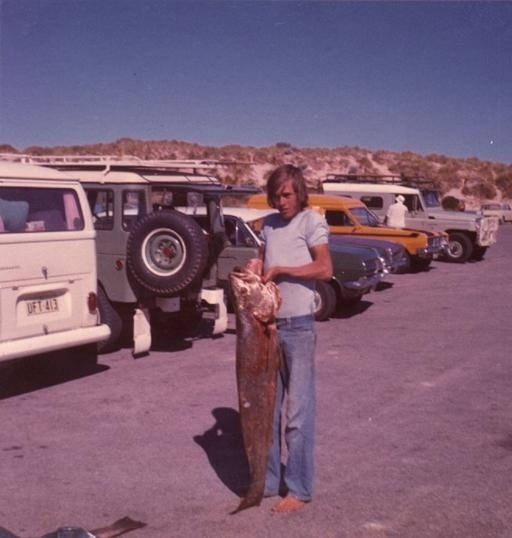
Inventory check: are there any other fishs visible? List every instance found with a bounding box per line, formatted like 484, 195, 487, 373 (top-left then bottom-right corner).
41, 517, 146, 538
227, 266, 282, 515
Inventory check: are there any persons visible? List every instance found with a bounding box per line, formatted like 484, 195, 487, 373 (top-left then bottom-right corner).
244, 164, 333, 514
384, 194, 409, 227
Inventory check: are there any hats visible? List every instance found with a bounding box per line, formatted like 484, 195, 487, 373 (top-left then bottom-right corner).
394, 195, 406, 204
